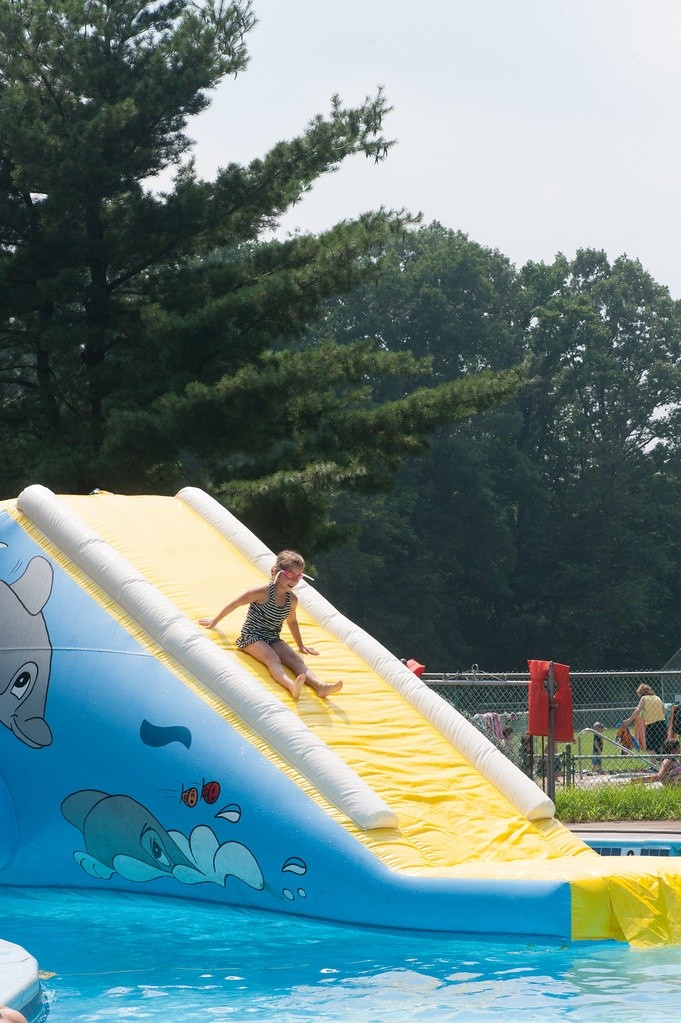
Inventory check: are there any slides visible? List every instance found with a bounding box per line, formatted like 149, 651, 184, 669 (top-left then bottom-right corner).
0, 481, 681, 944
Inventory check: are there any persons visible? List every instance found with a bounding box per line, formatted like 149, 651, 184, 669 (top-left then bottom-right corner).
519, 732, 561, 783
623, 683, 668, 772
199, 551, 343, 699
658, 703, 681, 780
590, 722, 604, 773
502, 727, 514, 749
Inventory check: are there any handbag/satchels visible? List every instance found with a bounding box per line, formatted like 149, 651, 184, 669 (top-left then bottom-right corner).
635, 696, 646, 750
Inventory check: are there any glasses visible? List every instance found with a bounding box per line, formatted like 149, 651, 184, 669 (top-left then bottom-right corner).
282, 569, 304, 581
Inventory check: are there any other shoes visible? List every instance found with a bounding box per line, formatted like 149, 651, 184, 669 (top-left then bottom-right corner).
648, 766, 657, 773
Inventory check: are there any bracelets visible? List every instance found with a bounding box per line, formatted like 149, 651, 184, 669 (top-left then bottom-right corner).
628, 718, 632, 722
